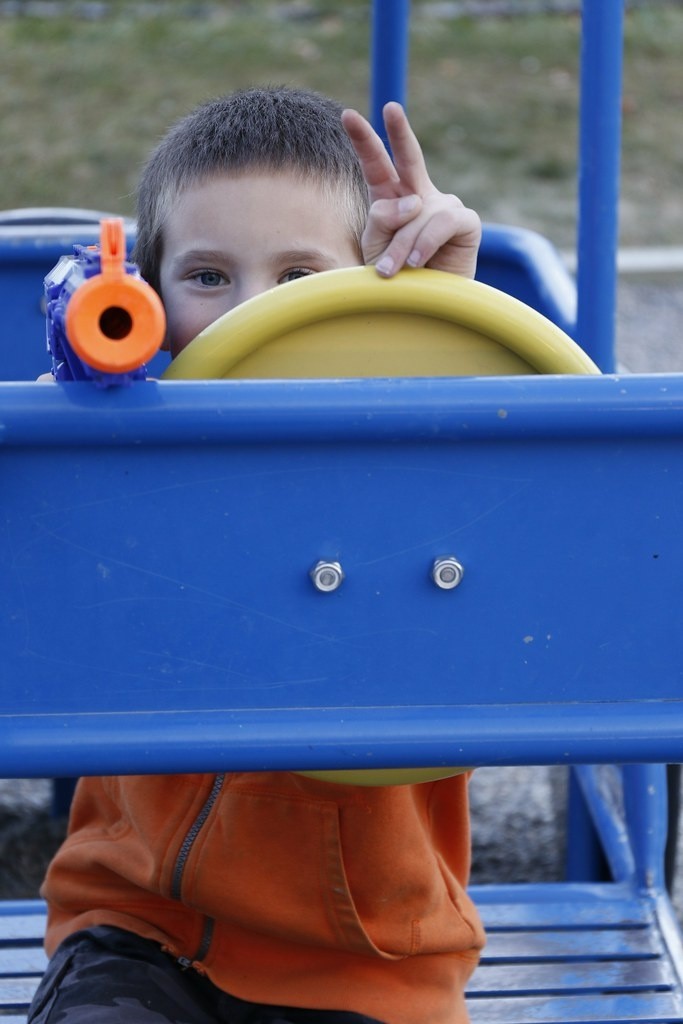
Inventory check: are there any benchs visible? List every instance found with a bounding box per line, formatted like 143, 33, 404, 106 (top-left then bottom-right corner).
0, 209, 683, 1024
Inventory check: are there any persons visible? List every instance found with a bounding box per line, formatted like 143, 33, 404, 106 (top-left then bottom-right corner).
24, 85, 491, 1024
